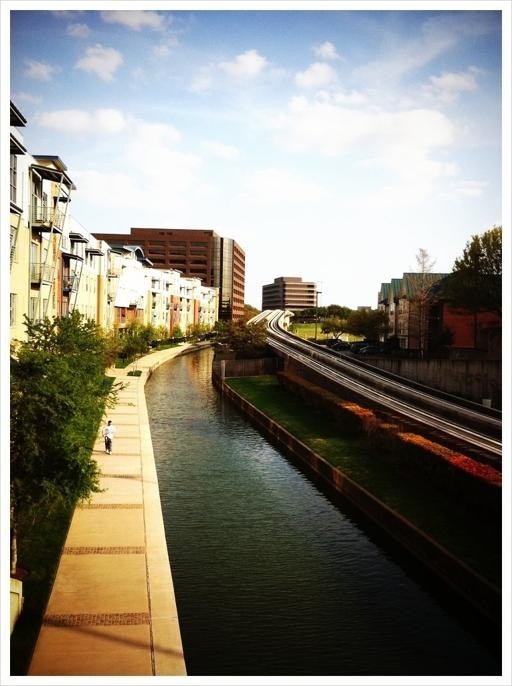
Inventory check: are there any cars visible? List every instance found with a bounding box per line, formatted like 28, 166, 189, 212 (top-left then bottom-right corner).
327, 339, 382, 356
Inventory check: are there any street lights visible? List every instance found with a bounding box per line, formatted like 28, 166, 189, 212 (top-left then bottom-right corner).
315, 290, 323, 340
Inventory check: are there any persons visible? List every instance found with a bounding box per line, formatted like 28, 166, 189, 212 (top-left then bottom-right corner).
102, 420, 113, 455
